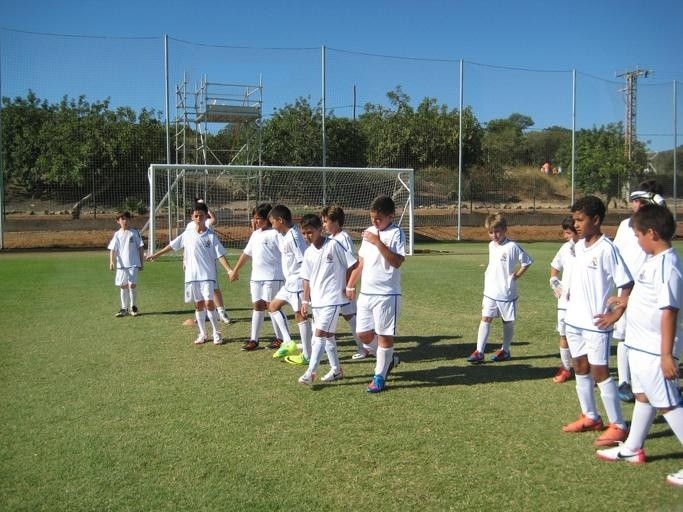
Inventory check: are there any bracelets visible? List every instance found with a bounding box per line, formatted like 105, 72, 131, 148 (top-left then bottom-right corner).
301, 301, 309, 304
346, 287, 355, 291
228, 269, 233, 275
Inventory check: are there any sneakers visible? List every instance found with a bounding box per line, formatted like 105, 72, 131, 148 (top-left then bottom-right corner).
321, 368, 343, 381
368, 374, 386, 392
565, 415, 605, 432
353, 347, 369, 359
298, 370, 317, 384
242, 340, 260, 351
264, 339, 284, 349
220, 312, 231, 324
213, 333, 224, 344
393, 351, 400, 365
273, 340, 299, 359
492, 349, 511, 361
286, 353, 309, 365
668, 468, 683, 483
595, 444, 646, 464
468, 351, 485, 362
595, 424, 628, 447
618, 383, 635, 401
194, 333, 207, 344
129, 306, 140, 316
554, 368, 574, 384
115, 308, 128, 317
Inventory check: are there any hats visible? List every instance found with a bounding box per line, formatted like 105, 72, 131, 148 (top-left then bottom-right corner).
630, 191, 653, 201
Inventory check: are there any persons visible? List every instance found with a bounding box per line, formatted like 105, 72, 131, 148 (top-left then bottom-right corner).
321, 205, 370, 360
467, 213, 534, 363
561, 195, 635, 447
228, 202, 287, 351
108, 211, 144, 317
550, 217, 581, 384
192, 208, 231, 325
345, 195, 406, 393
267, 204, 312, 366
596, 205, 683, 484
297, 214, 357, 385
612, 182, 668, 403
146, 203, 239, 344
